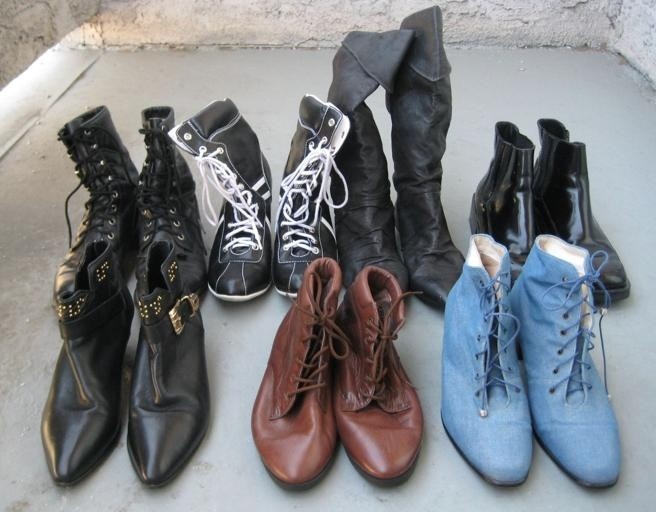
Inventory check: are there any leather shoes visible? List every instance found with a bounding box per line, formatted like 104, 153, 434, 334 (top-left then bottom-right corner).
530, 112, 634, 306
248, 253, 354, 496
437, 227, 535, 488
505, 230, 624, 493
462, 118, 538, 269
327, 260, 429, 489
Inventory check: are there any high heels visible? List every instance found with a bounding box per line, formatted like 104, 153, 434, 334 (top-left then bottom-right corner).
38, 237, 133, 489
122, 237, 215, 491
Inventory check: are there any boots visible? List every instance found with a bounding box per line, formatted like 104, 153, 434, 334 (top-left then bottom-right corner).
271, 90, 353, 303
134, 98, 206, 313
379, 4, 468, 313
326, 24, 417, 301
195, 305, 202, 307
52, 102, 138, 303
166, 95, 278, 305
